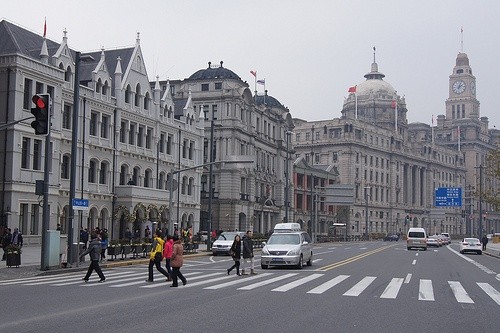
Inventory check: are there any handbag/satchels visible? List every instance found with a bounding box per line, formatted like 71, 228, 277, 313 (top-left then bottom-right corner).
228, 241, 236, 256
155, 252, 163, 263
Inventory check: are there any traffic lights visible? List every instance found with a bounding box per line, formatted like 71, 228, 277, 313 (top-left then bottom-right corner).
30, 93, 51, 138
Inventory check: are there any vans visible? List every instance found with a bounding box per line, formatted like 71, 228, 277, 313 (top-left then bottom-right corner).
405, 227, 429, 251
273, 222, 302, 232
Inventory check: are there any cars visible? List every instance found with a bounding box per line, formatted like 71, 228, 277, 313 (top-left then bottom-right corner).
212, 231, 247, 256
427, 233, 451, 248
260, 232, 314, 269
458, 237, 483, 255
383, 234, 399, 241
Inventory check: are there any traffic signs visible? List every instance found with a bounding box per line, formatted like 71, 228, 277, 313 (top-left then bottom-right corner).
434, 186, 463, 208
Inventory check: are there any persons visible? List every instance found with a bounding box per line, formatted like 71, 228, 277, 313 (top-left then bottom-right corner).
211, 227, 223, 247
1, 227, 23, 261
124, 227, 140, 242
482, 235, 488, 250
228, 235, 241, 276
174, 226, 191, 240
80, 234, 106, 282
80, 227, 89, 250
146, 230, 187, 287
57, 224, 63, 233
93, 228, 107, 262
242, 231, 256, 274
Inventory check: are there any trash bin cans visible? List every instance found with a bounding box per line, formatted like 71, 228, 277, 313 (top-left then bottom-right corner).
73, 242, 85, 262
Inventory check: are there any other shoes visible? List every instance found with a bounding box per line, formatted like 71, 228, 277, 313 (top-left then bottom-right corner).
227, 269, 230, 275
167, 273, 171, 281
251, 269, 257, 274
182, 280, 187, 285
146, 280, 153, 282
237, 273, 242, 276
98, 279, 105, 283
82, 277, 88, 282
170, 285, 178, 287
242, 270, 248, 274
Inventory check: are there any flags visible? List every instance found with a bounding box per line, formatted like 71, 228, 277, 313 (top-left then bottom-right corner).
257, 80, 264, 84
348, 86, 355, 92
250, 71, 256, 76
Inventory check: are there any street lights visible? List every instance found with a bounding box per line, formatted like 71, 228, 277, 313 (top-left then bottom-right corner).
206, 120, 224, 252
284, 131, 296, 223
66, 51, 96, 270
170, 159, 255, 231
473, 161, 488, 244
